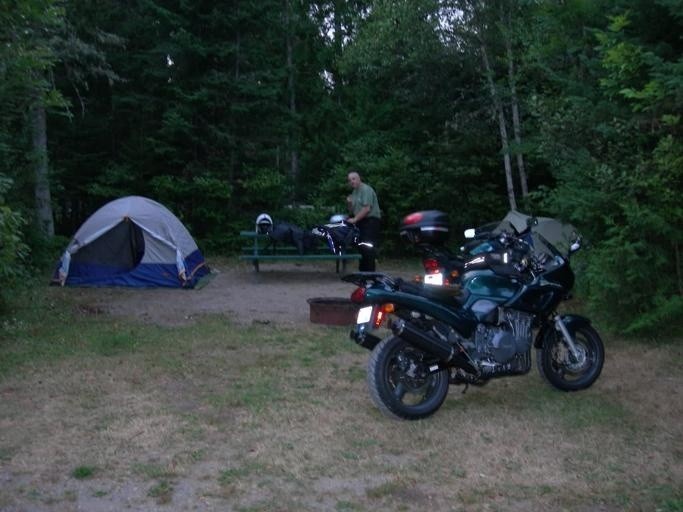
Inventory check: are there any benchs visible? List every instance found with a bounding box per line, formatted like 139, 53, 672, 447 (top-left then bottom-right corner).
237, 229, 362, 276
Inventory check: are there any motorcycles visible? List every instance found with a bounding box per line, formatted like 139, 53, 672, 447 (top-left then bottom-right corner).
340, 210, 604, 420
399, 210, 503, 285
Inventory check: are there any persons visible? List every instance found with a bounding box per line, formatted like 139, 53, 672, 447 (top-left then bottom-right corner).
346, 172, 381, 273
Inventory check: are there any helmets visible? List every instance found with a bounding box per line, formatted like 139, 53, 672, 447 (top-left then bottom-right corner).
256, 213, 273, 233
330, 215, 342, 223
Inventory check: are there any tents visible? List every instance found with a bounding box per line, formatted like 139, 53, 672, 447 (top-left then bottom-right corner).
46, 193, 208, 289
